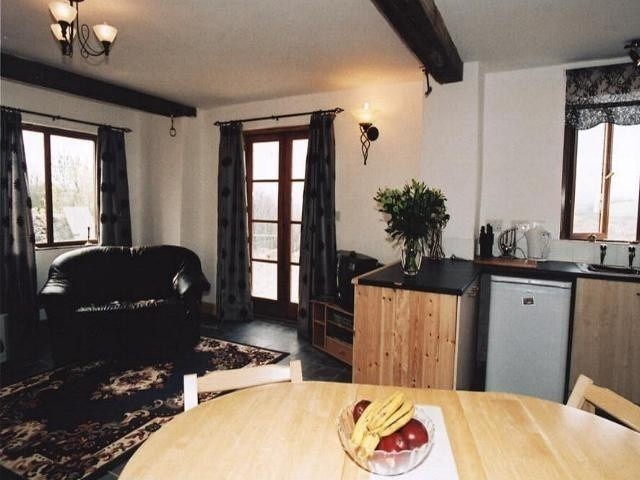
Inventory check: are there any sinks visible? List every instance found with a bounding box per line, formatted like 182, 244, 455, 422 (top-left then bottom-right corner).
575, 259, 639, 281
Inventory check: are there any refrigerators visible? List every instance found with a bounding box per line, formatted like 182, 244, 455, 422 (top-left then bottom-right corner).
484, 276, 573, 406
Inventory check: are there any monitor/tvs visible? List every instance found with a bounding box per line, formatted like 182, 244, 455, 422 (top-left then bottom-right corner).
334, 250, 382, 311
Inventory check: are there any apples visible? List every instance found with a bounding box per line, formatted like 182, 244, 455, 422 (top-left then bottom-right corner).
353, 400, 428, 470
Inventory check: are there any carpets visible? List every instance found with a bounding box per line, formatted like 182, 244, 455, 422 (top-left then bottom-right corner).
0, 336, 293, 480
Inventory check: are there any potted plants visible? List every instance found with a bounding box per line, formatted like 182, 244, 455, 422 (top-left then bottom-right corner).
372, 178, 450, 276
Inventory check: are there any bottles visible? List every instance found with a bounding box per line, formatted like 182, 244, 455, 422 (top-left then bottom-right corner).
479, 221, 493, 257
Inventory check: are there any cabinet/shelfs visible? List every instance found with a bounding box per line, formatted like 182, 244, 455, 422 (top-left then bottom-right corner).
565, 278, 640, 411
310, 295, 352, 366
352, 255, 482, 390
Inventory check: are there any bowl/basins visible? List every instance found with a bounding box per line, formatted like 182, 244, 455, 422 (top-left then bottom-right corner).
338, 401, 437, 476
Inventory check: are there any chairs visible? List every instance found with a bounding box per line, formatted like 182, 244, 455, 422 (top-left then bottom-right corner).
183, 360, 301, 413
563, 373, 640, 431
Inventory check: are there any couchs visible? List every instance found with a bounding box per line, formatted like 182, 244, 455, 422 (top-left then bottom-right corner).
37, 246, 211, 355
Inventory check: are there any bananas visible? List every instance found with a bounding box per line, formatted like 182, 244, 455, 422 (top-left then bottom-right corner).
349, 391, 416, 453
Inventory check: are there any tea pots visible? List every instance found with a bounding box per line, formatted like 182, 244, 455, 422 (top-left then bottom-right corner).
524, 226, 554, 263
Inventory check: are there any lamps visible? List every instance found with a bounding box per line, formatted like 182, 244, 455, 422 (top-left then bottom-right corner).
45, 1, 118, 58
350, 98, 381, 167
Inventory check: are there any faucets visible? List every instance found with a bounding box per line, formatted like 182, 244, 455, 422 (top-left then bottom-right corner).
599, 245, 606, 264
629, 245, 636, 265
